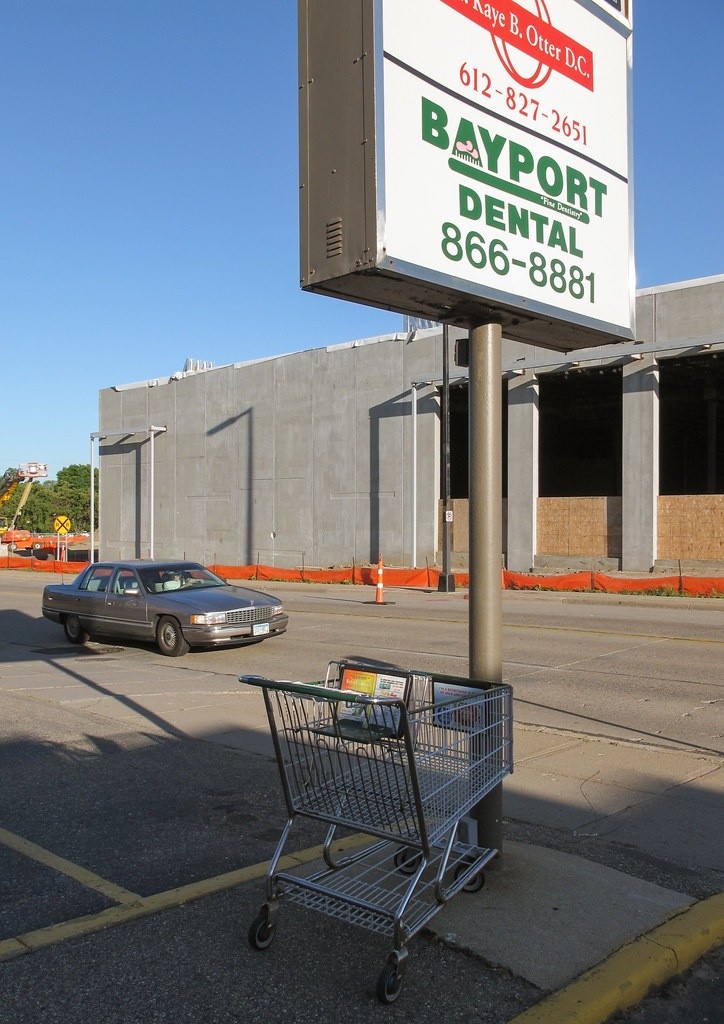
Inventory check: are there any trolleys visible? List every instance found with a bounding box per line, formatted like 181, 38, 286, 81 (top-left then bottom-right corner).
236, 658, 518, 1003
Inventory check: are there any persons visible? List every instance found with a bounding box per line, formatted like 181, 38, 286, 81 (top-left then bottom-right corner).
163, 570, 204, 592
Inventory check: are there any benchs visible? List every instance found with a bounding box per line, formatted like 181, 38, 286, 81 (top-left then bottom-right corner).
87, 576, 134, 593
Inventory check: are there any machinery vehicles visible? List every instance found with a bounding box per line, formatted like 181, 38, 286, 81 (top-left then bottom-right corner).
0, 463, 89, 551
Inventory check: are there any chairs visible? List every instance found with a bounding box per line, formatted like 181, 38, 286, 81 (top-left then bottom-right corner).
119, 580, 152, 594
155, 573, 172, 592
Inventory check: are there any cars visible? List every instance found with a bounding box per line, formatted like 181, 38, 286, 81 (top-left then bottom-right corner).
40, 559, 289, 658
